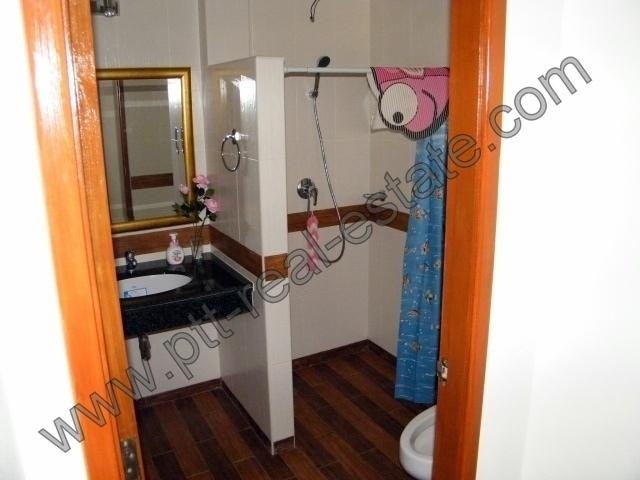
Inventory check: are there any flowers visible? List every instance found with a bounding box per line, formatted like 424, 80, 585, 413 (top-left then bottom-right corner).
182, 173, 218, 257
171, 183, 187, 215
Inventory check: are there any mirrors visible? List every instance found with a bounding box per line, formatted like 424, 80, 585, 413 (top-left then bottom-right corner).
96, 66, 200, 234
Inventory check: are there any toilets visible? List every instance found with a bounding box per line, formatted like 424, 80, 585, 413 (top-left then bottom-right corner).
398, 403, 436, 480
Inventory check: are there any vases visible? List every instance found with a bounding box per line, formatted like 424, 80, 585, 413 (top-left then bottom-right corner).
190, 237, 204, 262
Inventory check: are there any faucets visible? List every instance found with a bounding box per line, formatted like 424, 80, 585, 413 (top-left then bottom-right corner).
124, 250, 137, 271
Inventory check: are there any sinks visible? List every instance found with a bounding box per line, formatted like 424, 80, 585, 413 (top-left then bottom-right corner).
117, 268, 194, 299
